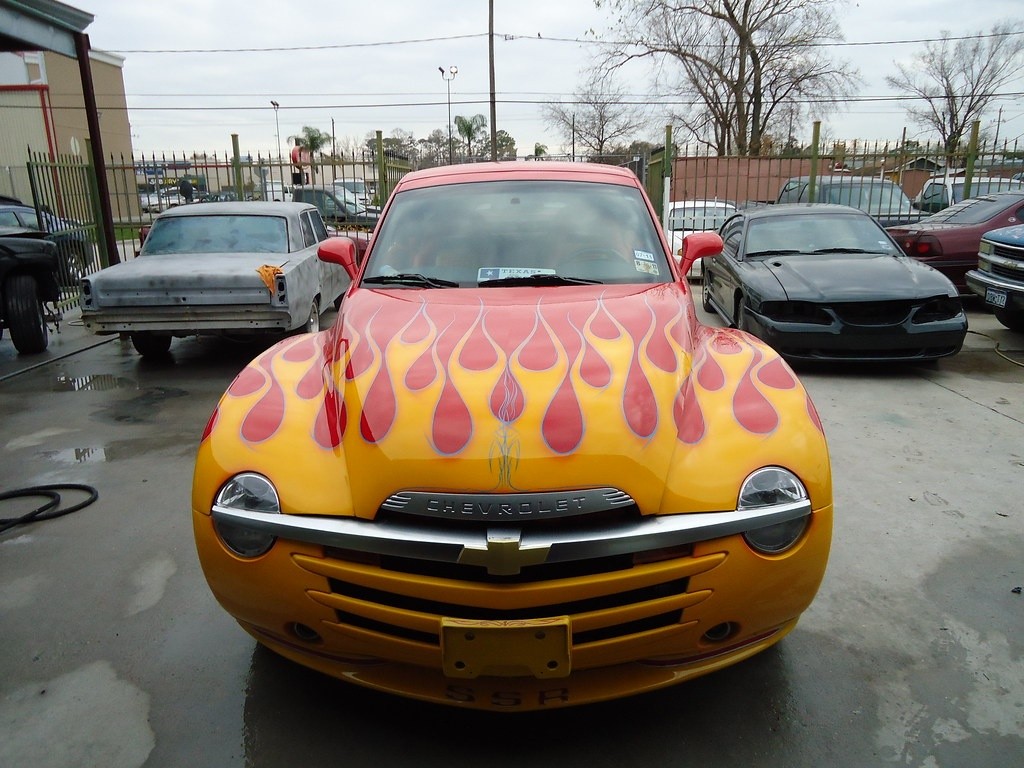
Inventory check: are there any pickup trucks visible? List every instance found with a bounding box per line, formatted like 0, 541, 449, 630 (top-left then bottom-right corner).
740, 174, 933, 228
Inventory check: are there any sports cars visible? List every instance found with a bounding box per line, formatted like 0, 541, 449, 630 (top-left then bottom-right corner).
192, 165, 834, 717
700, 202, 970, 368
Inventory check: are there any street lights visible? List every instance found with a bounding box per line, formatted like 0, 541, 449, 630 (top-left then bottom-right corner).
271, 99, 286, 202
439, 65, 459, 165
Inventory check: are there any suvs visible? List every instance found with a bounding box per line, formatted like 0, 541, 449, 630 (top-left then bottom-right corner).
332, 176, 376, 206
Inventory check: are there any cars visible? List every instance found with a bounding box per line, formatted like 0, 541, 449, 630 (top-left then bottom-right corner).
912, 177, 1024, 214
879, 189, 1024, 295
0, 235, 63, 356
966, 223, 1024, 334
76, 202, 351, 360
0, 194, 95, 286
668, 200, 745, 279
304, 221, 372, 264
140, 179, 383, 234
